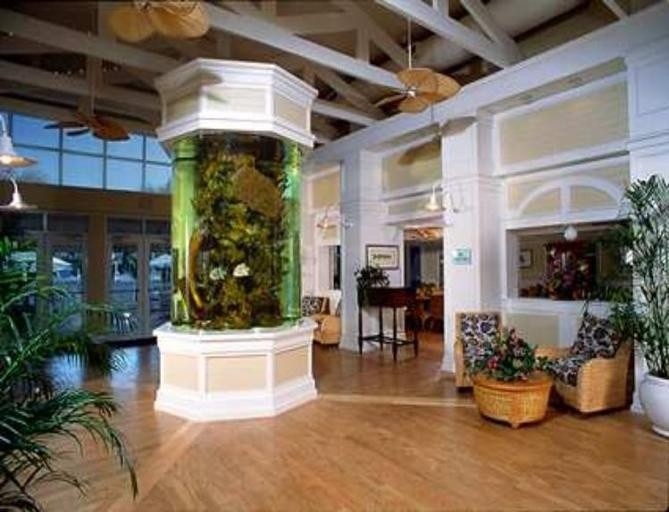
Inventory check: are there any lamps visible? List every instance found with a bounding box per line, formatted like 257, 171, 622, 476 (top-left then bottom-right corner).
426, 180, 456, 214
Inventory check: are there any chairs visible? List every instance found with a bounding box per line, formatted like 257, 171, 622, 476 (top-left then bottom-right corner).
302, 295, 342, 346
453, 309, 502, 388
532, 309, 634, 414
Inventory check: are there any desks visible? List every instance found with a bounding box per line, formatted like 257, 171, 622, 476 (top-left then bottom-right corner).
357, 286, 419, 362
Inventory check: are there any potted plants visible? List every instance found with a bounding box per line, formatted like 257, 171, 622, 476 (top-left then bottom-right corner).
577, 172, 668, 442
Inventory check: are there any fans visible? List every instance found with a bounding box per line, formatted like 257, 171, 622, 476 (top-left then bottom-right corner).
108, 1, 211, 45
43, 57, 130, 143
369, 16, 462, 115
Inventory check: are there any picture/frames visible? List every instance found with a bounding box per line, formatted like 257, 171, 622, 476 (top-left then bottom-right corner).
366, 242, 400, 271
517, 248, 533, 268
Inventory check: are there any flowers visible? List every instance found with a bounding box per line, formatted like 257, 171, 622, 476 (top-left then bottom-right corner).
475, 327, 539, 381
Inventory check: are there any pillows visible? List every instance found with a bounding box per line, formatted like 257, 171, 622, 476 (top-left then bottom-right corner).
459, 313, 500, 347
570, 311, 626, 361
